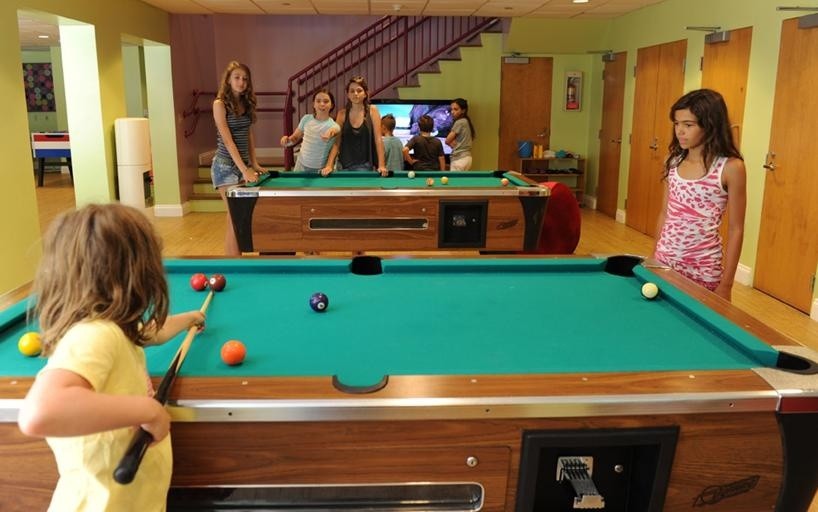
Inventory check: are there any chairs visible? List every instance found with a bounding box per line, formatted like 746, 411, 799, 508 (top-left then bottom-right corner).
32, 131, 74, 186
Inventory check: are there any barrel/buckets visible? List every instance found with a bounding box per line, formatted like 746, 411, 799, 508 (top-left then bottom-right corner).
518, 140, 532, 158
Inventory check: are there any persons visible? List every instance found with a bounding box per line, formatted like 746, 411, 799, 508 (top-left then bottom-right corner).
444, 97, 476, 171
649, 88, 747, 303
320, 75, 389, 177
402, 114, 446, 171
279, 89, 341, 172
208, 61, 268, 255
380, 112, 405, 171
15, 204, 205, 512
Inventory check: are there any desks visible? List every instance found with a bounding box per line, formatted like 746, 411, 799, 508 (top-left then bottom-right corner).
225, 170, 552, 253
0, 251, 818, 512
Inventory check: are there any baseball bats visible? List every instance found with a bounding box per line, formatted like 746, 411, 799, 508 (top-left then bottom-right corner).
112, 291, 215, 484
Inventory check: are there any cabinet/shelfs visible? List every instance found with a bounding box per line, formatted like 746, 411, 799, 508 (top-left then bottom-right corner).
520, 157, 583, 203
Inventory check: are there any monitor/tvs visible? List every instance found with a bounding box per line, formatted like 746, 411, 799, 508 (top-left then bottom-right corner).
369, 99, 467, 164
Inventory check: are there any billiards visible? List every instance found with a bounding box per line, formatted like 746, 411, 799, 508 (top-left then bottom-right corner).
309, 293, 328, 311
501, 178, 509, 185
191, 272, 207, 290
408, 171, 449, 188
208, 274, 225, 290
642, 281, 659, 299
221, 340, 245, 364
18, 333, 43, 356
